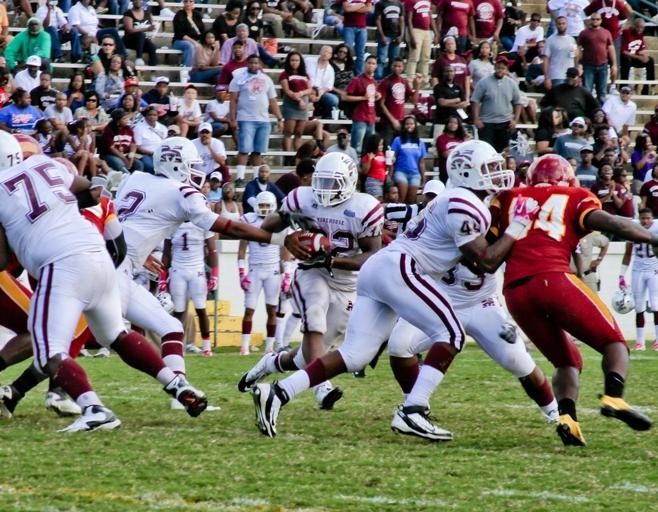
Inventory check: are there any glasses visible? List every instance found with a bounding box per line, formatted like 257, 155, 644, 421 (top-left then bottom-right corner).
103, 42, 116, 47
572, 123, 584, 129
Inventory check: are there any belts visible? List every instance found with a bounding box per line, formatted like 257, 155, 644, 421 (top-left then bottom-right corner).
583, 266, 596, 276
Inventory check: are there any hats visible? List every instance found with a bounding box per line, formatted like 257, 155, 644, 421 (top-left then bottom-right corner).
423, 179, 445, 195
579, 145, 594, 154
198, 122, 213, 133
26, 55, 42, 67
568, 117, 588, 132
336, 128, 349, 136
156, 76, 169, 84
124, 78, 139, 87
565, 67, 578, 75
495, 55, 509, 64
620, 86, 632, 94
210, 171, 223, 182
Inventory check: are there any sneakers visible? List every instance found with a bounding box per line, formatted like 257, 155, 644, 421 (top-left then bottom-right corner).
389, 404, 454, 442
237, 351, 286, 393
631, 343, 646, 352
543, 408, 562, 426
170, 398, 186, 410
251, 379, 289, 438
0, 386, 23, 420
56, 404, 122, 435
92, 346, 111, 359
162, 374, 209, 419
46, 388, 82, 416
186, 342, 202, 353
649, 341, 658, 352
314, 384, 344, 410
556, 414, 586, 448
598, 391, 654, 432
238, 346, 250, 357
202, 348, 213, 358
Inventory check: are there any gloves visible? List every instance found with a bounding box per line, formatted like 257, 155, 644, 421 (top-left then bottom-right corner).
207, 267, 219, 292
157, 270, 169, 292
296, 256, 335, 280
280, 272, 294, 293
237, 267, 252, 292
505, 196, 541, 239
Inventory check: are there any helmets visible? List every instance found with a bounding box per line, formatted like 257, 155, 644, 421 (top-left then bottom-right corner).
152, 135, 206, 190
156, 291, 176, 314
252, 191, 277, 217
446, 139, 515, 191
526, 154, 579, 186
312, 151, 358, 207
12, 133, 43, 161
612, 290, 637, 315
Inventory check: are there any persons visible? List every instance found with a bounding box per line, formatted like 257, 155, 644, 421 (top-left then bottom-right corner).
99, 35, 117, 74
96, 53, 126, 112
215, 181, 245, 241
555, 117, 593, 165
545, 0, 591, 37
486, 155, 658, 445
597, 129, 629, 162
1, 66, 12, 109
145, 77, 183, 127
238, 149, 385, 410
108, 94, 140, 125
197, 180, 211, 209
133, 109, 168, 173
304, 45, 340, 120
382, 59, 422, 140
592, 111, 620, 161
221, 24, 260, 64
247, 2, 265, 45
107, 138, 271, 409
430, 36, 471, 96
191, 33, 225, 85
432, 67, 468, 123
591, 165, 626, 216
639, 165, 658, 212
598, 149, 625, 184
208, 171, 226, 203
632, 133, 658, 193
77, 91, 109, 131
31, 73, 57, 110
341, 56, 382, 148
615, 168, 637, 219
442, 1, 477, 53
172, 1, 207, 67
264, 1, 344, 39
473, 55, 523, 152
407, 1, 438, 91
0, 157, 127, 416
477, 1, 504, 44
4, 17, 53, 82
644, 104, 658, 148
508, 12, 544, 76
238, 192, 293, 355
67, 1, 100, 53
66, 72, 87, 116
74, 117, 98, 178
618, 208, 658, 350
526, 40, 546, 86
0, 132, 58, 376
566, 158, 578, 174
66, 120, 111, 170
362, 137, 397, 205
1, 131, 208, 435
570, 233, 610, 300
329, 46, 356, 121
162, 216, 219, 361
328, 130, 360, 170
121, 77, 152, 110
230, 56, 287, 185
389, 182, 559, 422
250, 140, 541, 442
600, 88, 638, 137
44, 92, 74, 133
540, 68, 600, 117
208, 87, 236, 138
0, 87, 45, 133
623, 18, 656, 92
125, 1, 156, 69
376, 1, 406, 80
282, 51, 308, 150
542, 16, 581, 89
469, 45, 494, 83
438, 118, 470, 182
35, 1, 84, 62
585, 2, 633, 81
175, 85, 203, 136
577, 147, 599, 190
36, 120, 58, 153
14, 55, 42, 91
346, 1, 375, 73
220, 40, 252, 84
102, 110, 143, 171
390, 115, 428, 204
575, 15, 617, 97
211, 0, 246, 47
189, 124, 231, 180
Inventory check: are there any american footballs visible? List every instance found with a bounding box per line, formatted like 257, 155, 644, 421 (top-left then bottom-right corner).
297, 231, 330, 258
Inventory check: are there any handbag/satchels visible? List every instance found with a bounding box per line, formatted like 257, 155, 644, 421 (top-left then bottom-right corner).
86, 98, 97, 103
